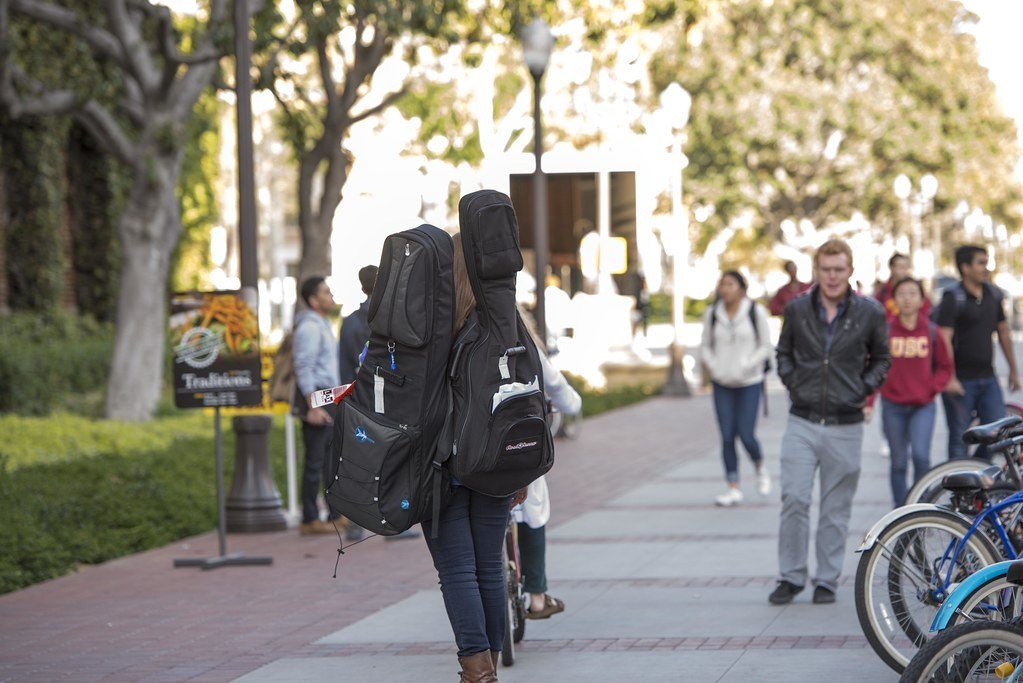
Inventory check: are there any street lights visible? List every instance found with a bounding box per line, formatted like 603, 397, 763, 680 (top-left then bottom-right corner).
515, 14, 555, 355
658, 82, 693, 394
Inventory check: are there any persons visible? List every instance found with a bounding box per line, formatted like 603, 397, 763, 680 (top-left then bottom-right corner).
768, 254, 929, 455
880, 277, 953, 509
417, 232, 584, 682
292, 265, 419, 540
699, 270, 773, 507
766, 240, 891, 603
932, 245, 1020, 462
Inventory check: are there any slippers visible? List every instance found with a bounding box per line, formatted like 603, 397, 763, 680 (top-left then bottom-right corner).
526, 594, 565, 619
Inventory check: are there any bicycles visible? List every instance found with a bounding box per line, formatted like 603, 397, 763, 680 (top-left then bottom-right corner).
856, 415, 1023, 683
499, 512, 549, 667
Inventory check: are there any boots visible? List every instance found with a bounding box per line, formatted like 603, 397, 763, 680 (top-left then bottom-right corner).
457, 649, 499, 683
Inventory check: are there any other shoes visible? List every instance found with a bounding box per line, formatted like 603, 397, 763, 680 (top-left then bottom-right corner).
755, 471, 769, 494
299, 518, 342, 536
327, 515, 348, 526
768, 580, 805, 604
714, 487, 742, 505
814, 584, 836, 603
